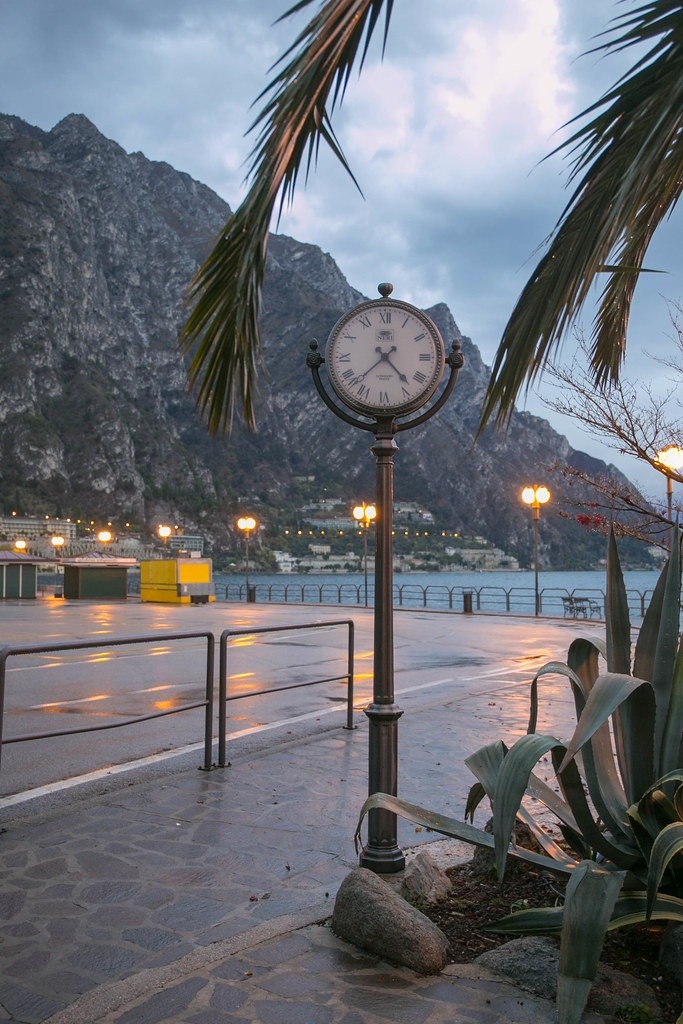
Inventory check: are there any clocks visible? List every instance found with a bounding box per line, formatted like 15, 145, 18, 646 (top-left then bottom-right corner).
328, 302, 441, 412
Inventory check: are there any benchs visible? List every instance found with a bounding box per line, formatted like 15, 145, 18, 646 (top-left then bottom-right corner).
561, 596, 602, 620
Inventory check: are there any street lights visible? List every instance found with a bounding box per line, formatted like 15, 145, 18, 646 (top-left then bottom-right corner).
652, 443, 683, 559
521, 484, 551, 615
51, 535, 65, 585
158, 526, 172, 560
352, 501, 377, 606
236, 516, 256, 603
99, 531, 112, 553
